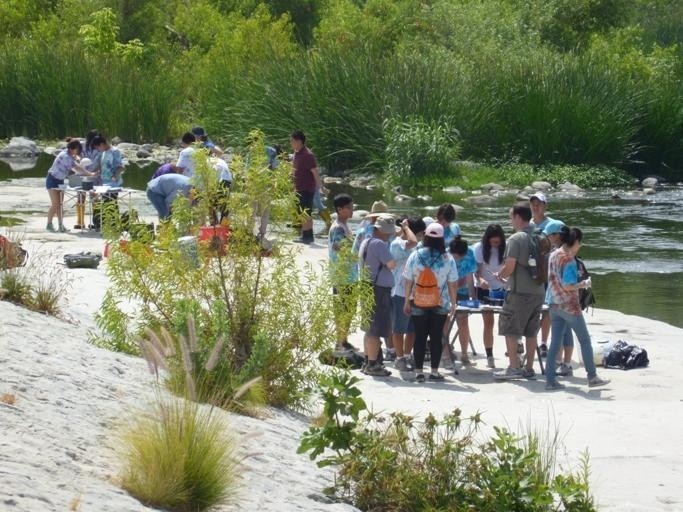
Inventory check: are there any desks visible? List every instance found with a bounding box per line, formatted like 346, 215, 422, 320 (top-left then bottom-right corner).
443, 303, 545, 376
49, 186, 135, 235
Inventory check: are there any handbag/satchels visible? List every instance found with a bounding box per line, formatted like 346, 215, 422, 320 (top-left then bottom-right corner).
602, 338, 649, 369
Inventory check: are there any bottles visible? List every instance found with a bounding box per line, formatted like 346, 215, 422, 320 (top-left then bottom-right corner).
527, 255, 538, 279
456, 299, 479, 307
103, 243, 108, 257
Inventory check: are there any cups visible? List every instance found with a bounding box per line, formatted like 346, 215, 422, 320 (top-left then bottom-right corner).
64, 179, 68, 186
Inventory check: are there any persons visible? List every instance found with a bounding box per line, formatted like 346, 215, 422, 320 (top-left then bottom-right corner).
48, 130, 125, 232
146, 128, 278, 255
291, 130, 333, 244
328, 193, 611, 390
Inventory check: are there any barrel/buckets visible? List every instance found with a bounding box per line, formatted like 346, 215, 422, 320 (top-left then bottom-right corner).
82, 180, 94, 190
193, 224, 231, 257
577, 342, 615, 367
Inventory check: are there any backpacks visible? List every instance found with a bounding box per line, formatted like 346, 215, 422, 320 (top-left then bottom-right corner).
413, 266, 441, 308
575, 259, 596, 310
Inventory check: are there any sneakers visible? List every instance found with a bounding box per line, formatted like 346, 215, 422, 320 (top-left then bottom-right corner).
487, 344, 536, 379
539, 343, 611, 390
360, 350, 445, 382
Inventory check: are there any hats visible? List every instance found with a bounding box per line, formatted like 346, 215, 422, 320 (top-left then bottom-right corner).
365, 200, 402, 233
192, 127, 208, 138
530, 191, 566, 236
422, 216, 444, 238
